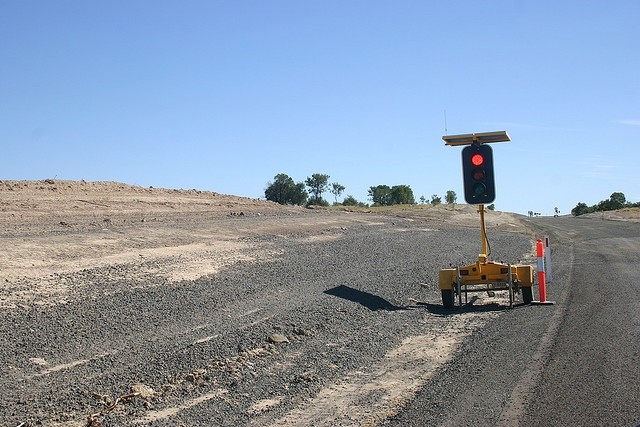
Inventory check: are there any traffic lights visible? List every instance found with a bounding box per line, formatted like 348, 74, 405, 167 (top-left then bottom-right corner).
461, 143, 495, 204
